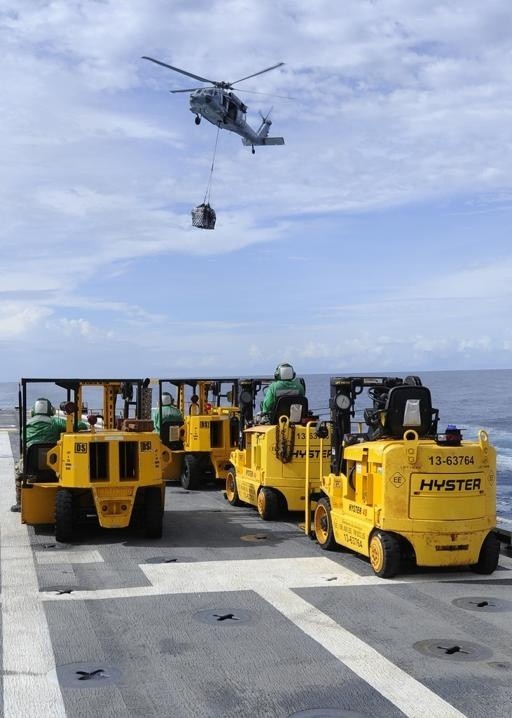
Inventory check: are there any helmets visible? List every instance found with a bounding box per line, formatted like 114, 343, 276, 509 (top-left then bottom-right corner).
31, 398, 53, 417
162, 392, 172, 406
275, 364, 296, 380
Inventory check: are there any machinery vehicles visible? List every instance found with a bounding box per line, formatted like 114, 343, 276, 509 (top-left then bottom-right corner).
219, 379, 332, 520
13, 377, 163, 542
304, 374, 502, 577
160, 377, 243, 490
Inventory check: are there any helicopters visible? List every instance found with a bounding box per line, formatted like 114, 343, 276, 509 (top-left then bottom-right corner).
141, 53, 287, 155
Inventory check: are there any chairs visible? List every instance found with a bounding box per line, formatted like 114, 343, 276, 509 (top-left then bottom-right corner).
383, 385, 432, 441
270, 395, 309, 425
26, 442, 59, 483
161, 419, 184, 450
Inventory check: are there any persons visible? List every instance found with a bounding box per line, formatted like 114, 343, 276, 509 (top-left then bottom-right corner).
152, 392, 182, 432
10, 398, 87, 512
261, 362, 305, 417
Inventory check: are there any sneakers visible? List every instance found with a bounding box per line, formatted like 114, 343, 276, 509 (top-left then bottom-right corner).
11, 505, 22, 512
